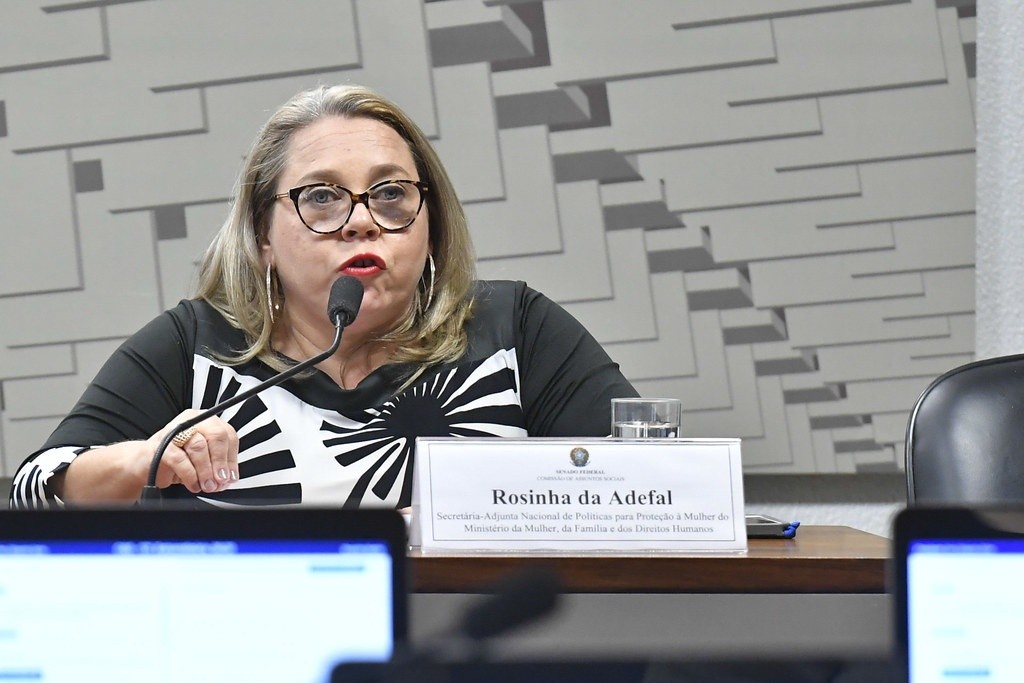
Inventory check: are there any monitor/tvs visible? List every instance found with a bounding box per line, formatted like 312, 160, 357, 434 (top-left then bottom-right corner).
888, 501, 1024, 683
0, 503, 412, 683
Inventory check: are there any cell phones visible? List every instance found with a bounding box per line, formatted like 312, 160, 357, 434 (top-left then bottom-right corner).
744, 513, 784, 539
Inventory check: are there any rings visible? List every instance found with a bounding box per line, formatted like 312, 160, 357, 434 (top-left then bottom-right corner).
172, 426, 198, 447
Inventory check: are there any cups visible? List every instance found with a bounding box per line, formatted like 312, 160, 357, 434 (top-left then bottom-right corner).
609, 395, 684, 440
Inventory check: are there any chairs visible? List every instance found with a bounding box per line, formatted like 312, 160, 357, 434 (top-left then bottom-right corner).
906, 352, 1024, 505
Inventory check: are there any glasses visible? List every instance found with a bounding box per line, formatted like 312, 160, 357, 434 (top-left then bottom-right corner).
269, 180, 428, 233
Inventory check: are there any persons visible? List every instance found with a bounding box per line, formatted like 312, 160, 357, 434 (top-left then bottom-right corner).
7, 86, 663, 510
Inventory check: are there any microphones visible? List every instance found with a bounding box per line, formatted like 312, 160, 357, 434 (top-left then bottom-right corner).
135, 274, 368, 504
381, 565, 560, 666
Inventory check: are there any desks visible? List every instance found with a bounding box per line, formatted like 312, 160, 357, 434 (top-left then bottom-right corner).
403, 527, 895, 595
